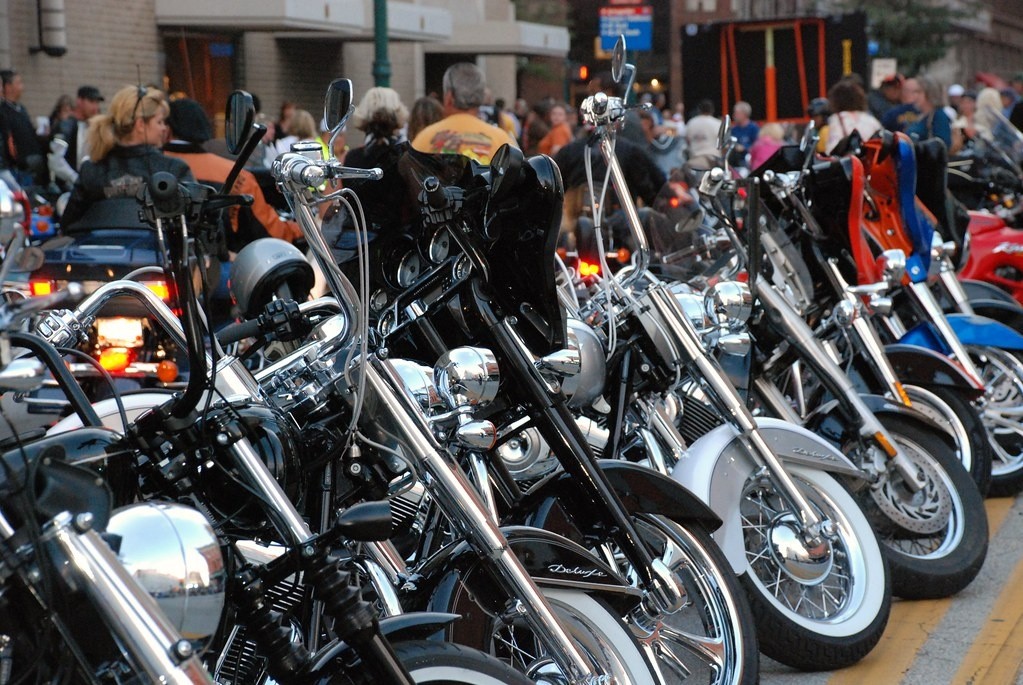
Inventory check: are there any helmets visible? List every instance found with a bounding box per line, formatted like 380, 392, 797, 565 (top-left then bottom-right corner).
230, 237, 316, 319
807, 97, 832, 117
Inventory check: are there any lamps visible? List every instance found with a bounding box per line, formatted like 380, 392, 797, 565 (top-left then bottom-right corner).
26, 0, 68, 58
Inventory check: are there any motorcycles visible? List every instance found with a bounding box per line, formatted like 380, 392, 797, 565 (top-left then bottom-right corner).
0, 34, 1023, 685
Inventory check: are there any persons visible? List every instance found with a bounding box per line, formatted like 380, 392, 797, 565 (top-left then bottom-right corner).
158, 98, 304, 254
336, 86, 409, 229
637, 92, 790, 181
477, 374, 483, 385
411, 62, 515, 166
407, 96, 448, 142
477, 87, 578, 156
60, 85, 195, 238
45, 85, 106, 192
0, 69, 42, 171
203, 93, 349, 191
806, 73, 1023, 163
718, 300, 723, 309
587, 71, 646, 147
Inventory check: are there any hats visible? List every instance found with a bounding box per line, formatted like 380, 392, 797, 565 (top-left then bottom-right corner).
78, 86, 104, 101
947, 83, 964, 98
168, 99, 213, 143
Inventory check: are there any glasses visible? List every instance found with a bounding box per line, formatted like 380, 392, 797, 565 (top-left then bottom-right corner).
132, 85, 145, 119
